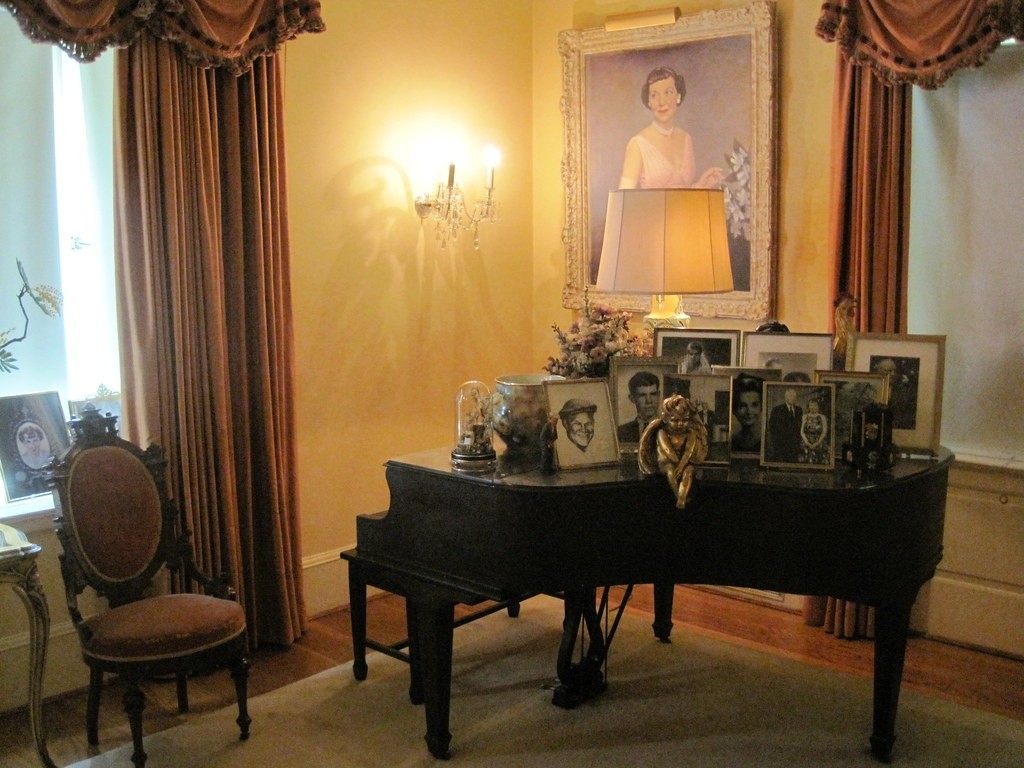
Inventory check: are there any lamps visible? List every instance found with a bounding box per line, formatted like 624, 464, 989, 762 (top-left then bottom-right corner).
414, 144, 494, 250
597, 185, 731, 327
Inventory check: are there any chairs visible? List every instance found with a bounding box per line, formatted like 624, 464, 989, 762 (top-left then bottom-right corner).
41, 398, 253, 768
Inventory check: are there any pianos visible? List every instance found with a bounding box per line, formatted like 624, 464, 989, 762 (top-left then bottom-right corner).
355, 441, 956, 762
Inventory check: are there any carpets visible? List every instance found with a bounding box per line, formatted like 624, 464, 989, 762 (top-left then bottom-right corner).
60, 597, 1024, 768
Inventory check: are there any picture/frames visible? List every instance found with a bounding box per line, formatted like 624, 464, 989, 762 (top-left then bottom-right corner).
543, 326, 947, 475
556, 3, 776, 324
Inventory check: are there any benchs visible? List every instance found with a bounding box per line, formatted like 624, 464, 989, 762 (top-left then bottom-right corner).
340, 548, 520, 705
